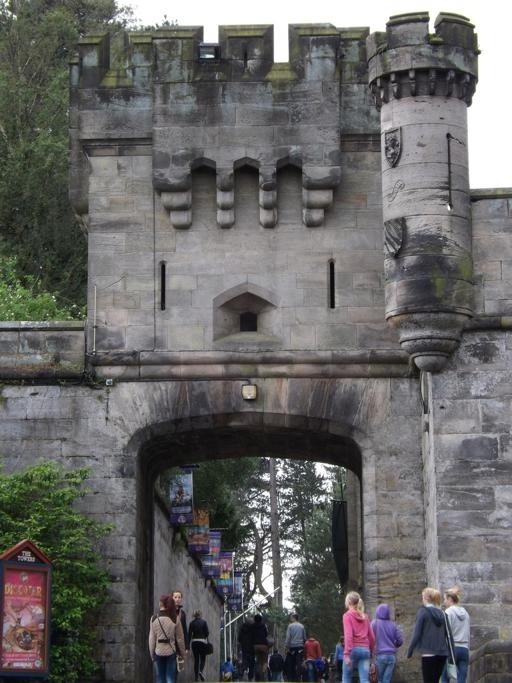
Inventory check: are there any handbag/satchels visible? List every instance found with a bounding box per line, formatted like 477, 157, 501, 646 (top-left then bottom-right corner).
175, 654, 185, 673
369, 664, 377, 682
204, 642, 214, 654
447, 663, 457, 679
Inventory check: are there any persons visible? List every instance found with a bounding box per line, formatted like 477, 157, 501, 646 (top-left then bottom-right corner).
222, 647, 330, 683
237, 618, 255, 681
305, 635, 323, 683
249, 614, 268, 682
172, 592, 191, 651
340, 590, 375, 683
440, 587, 471, 683
335, 635, 344, 683
149, 593, 186, 683
369, 603, 404, 683
284, 611, 306, 683
187, 611, 211, 681
406, 588, 455, 683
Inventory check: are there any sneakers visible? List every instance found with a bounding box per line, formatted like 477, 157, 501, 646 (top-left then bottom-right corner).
195, 672, 205, 682
